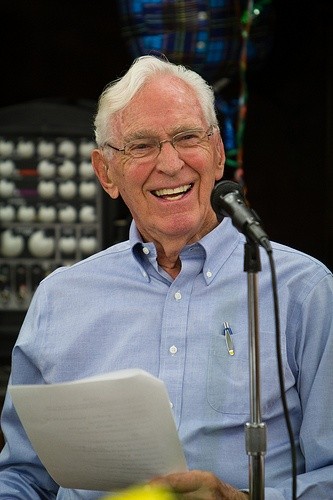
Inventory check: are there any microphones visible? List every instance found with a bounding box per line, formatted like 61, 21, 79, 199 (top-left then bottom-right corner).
210, 180, 272, 254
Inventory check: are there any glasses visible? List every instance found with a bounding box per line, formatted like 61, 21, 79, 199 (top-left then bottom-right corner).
106, 125, 213, 160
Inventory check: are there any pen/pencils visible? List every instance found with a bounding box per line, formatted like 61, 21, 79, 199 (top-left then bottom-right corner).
223, 321, 235, 356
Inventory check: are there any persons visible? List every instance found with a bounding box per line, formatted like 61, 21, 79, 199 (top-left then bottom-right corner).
0, 55, 333, 500
